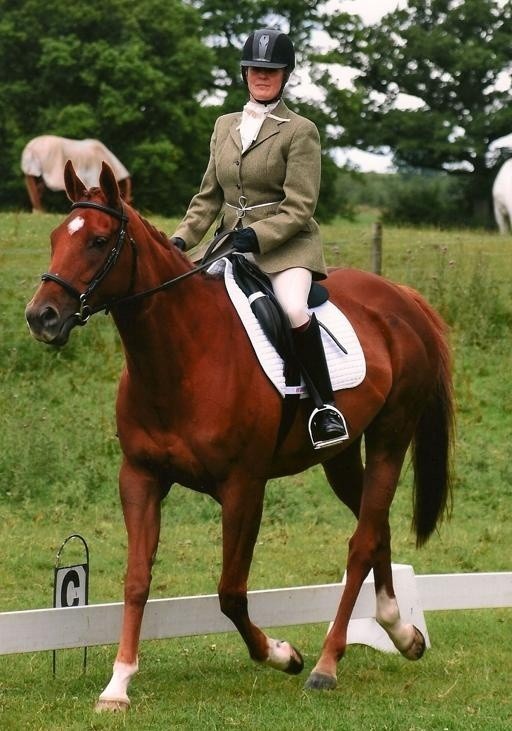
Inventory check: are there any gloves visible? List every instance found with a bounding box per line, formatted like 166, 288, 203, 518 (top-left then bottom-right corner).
229, 227, 259, 254
169, 237, 185, 252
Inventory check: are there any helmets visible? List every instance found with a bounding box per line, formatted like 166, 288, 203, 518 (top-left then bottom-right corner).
240, 29, 295, 69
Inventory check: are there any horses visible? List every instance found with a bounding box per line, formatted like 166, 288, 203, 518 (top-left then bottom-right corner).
23, 158, 459, 717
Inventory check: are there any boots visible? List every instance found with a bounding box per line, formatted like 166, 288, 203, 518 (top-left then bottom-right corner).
288, 312, 349, 449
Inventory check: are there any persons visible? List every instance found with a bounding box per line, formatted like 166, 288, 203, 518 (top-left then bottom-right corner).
162, 29, 351, 457
